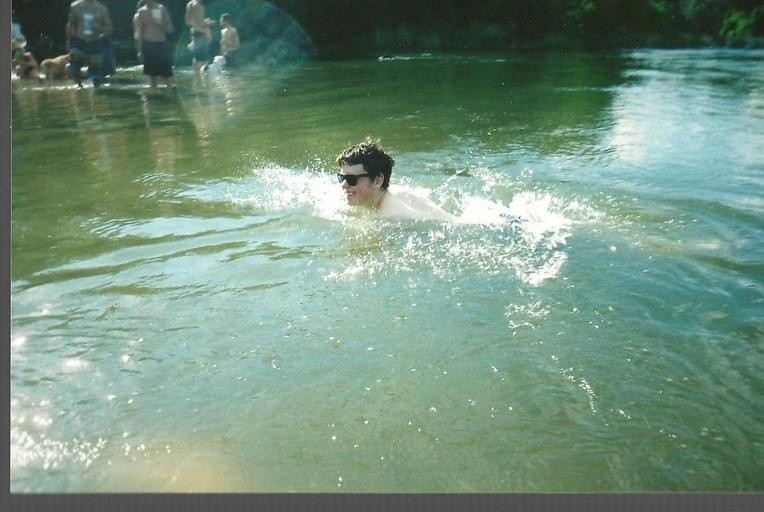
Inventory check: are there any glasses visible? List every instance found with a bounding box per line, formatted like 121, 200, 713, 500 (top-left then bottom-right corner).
336, 172, 370, 188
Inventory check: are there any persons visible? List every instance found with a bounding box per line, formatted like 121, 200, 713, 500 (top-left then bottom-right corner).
334, 142, 419, 218
11, 0, 241, 92
